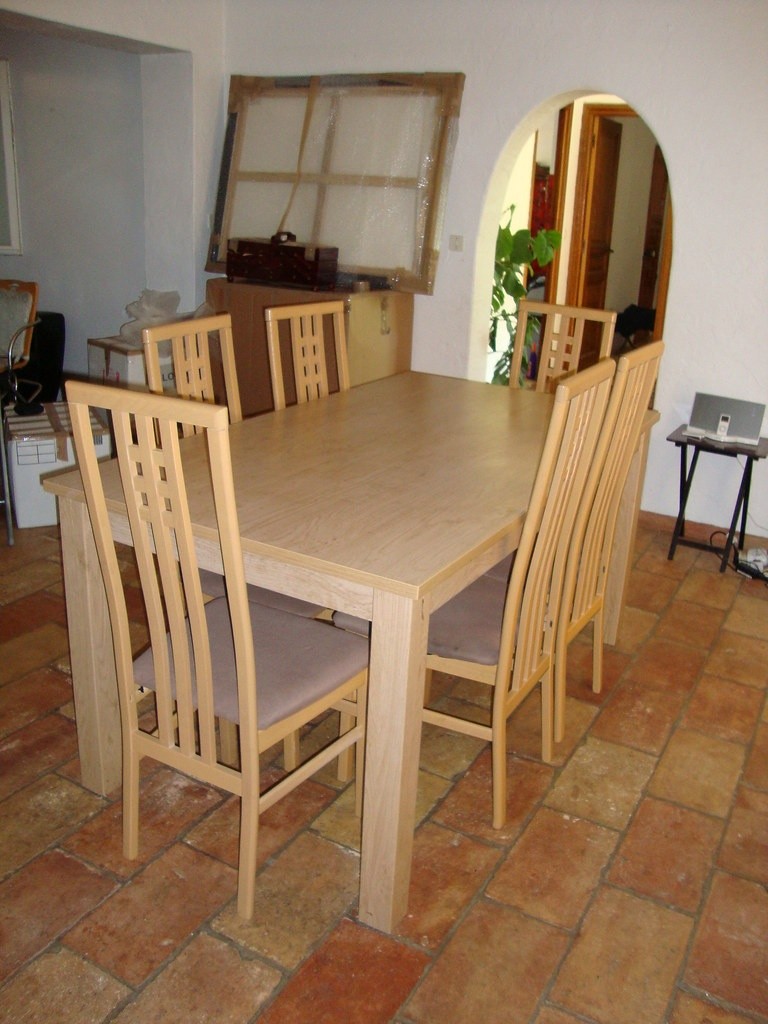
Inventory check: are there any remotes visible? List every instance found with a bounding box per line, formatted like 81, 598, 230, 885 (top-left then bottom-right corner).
716, 414, 730, 436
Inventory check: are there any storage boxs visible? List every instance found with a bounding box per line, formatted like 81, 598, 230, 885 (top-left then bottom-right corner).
87, 334, 178, 395
3, 402, 112, 530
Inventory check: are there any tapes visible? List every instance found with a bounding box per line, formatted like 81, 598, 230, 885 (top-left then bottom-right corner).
353, 281, 370, 293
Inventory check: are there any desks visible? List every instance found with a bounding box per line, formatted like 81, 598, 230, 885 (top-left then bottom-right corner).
664, 421, 767, 573
42, 369, 661, 937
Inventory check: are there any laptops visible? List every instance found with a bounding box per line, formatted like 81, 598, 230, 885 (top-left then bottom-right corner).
680, 391, 767, 445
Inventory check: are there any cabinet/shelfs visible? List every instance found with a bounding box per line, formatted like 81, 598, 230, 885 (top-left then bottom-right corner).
203, 277, 416, 419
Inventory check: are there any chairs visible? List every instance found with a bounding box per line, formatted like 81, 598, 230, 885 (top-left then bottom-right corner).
63, 379, 373, 924
0, 278, 47, 410
141, 299, 667, 830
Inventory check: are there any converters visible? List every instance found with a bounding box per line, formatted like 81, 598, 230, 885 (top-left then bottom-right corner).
735, 563, 763, 579
